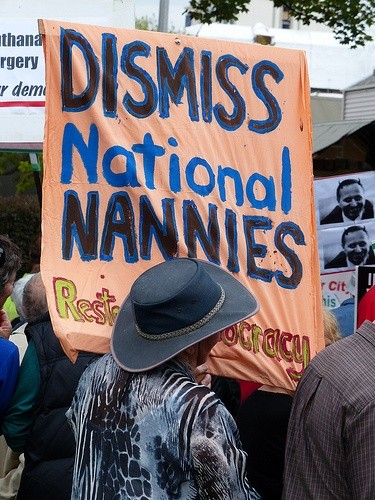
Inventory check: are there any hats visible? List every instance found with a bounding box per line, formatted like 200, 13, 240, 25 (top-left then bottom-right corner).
110, 258, 260, 372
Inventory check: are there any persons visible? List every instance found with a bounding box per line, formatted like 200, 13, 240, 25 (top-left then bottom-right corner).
0, 233, 374, 500
322, 226, 374, 268
320, 177, 375, 225
